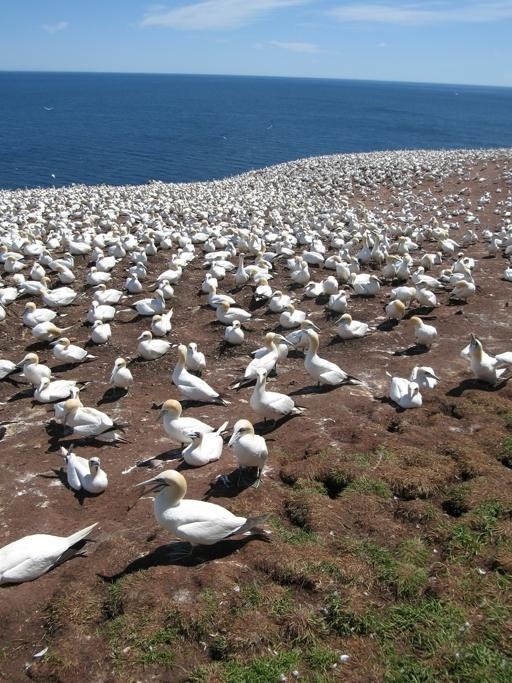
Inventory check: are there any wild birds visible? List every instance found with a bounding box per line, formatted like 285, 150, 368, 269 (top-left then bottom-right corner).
0, 521, 103, 584
50, 446, 108, 495
181, 420, 229, 467
227, 418, 268, 489
0, 147, 512, 444
135, 468, 271, 563
